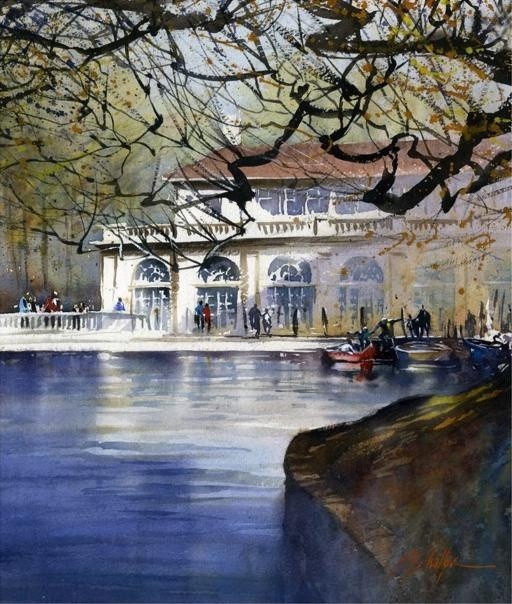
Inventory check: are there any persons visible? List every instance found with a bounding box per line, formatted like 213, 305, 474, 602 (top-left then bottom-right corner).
19, 289, 126, 330
345, 304, 512, 352
195, 300, 271, 338
